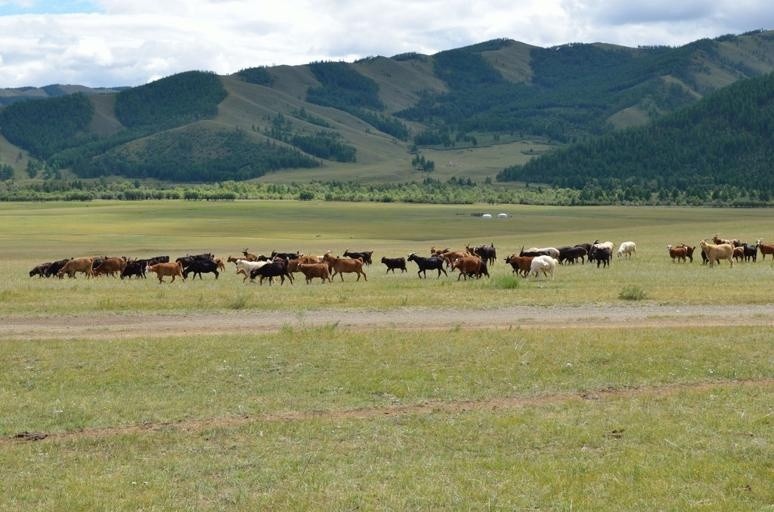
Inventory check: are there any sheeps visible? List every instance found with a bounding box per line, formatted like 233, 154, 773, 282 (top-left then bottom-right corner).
381, 256, 407, 275
407, 252, 448, 279
665, 235, 774, 269
503, 239, 615, 281
616, 241, 637, 261
431, 240, 496, 281
29, 248, 374, 287
